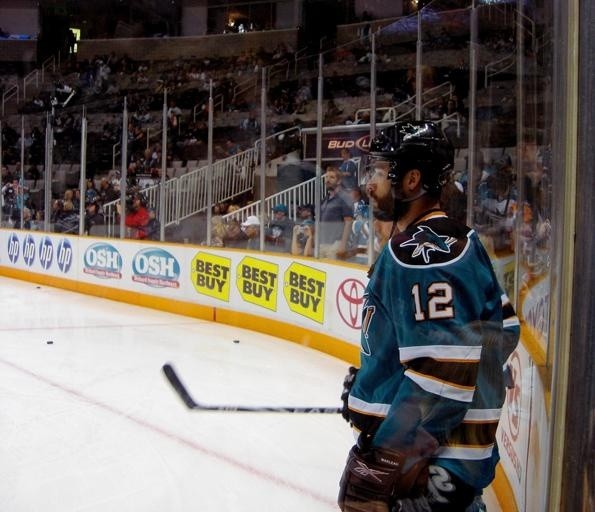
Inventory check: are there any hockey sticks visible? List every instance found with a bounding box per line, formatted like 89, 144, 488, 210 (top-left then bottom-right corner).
163, 365, 344, 414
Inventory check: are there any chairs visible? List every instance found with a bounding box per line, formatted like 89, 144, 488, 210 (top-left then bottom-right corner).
3, 164, 78, 200
452, 147, 518, 173
86, 108, 192, 141
5, 113, 44, 136
214, 96, 391, 131
91, 159, 226, 192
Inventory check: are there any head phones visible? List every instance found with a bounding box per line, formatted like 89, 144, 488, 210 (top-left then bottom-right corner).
141, 194, 147, 206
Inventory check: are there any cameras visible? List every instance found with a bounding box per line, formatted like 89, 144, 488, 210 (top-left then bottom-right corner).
58, 200, 64, 208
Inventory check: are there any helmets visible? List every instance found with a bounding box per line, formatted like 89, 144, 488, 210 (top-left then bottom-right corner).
367, 121, 455, 204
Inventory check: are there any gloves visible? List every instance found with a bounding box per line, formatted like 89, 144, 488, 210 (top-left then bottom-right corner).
340, 367, 360, 423
337, 430, 406, 512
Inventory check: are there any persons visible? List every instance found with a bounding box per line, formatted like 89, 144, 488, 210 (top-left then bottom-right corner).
336, 120, 520, 512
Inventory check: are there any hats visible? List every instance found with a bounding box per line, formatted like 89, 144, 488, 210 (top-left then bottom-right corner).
270, 204, 288, 214
241, 216, 260, 227
